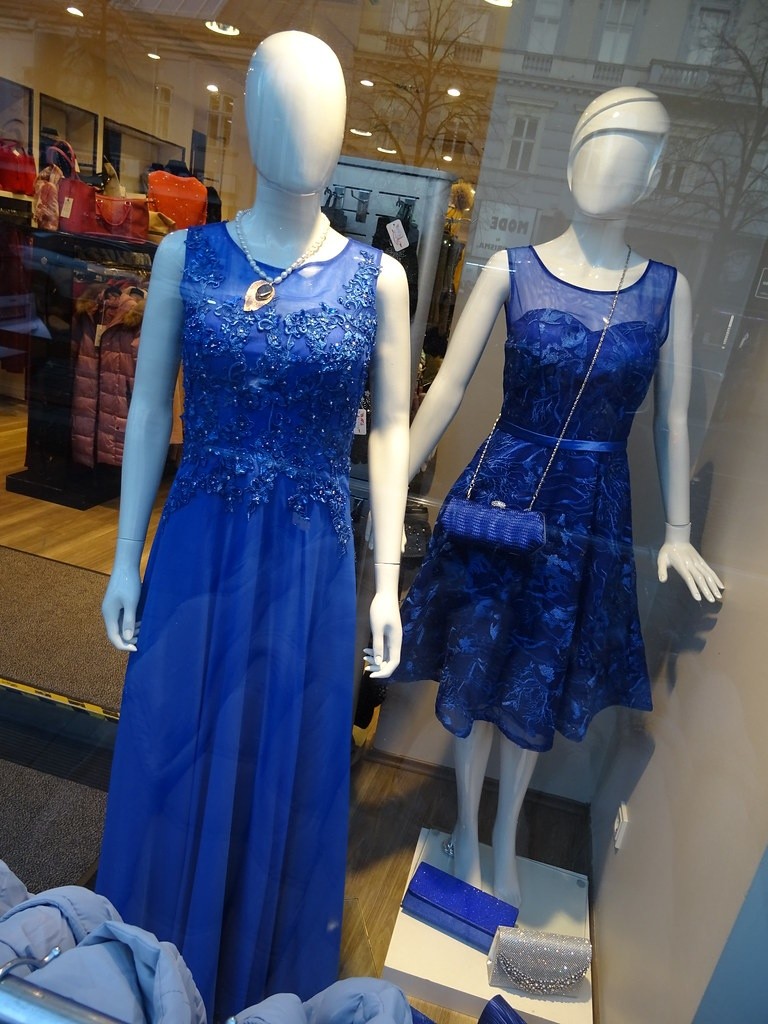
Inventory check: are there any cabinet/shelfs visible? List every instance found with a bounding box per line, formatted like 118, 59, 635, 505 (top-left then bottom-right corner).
0, 29, 194, 412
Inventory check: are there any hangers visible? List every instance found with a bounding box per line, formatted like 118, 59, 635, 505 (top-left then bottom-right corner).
73, 245, 152, 301
322, 189, 425, 223
443, 217, 464, 248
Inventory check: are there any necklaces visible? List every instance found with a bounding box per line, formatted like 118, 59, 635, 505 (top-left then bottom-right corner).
236, 208, 331, 311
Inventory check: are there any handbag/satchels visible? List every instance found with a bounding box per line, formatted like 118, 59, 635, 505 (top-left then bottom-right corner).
0, 117, 181, 246
439, 497, 547, 558
486, 925, 594, 998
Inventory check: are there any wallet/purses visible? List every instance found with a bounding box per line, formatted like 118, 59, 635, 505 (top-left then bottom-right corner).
399, 861, 520, 956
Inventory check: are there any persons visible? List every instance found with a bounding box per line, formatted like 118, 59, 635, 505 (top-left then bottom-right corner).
147, 160, 208, 233
95, 30, 410, 1024
365, 86, 727, 908
